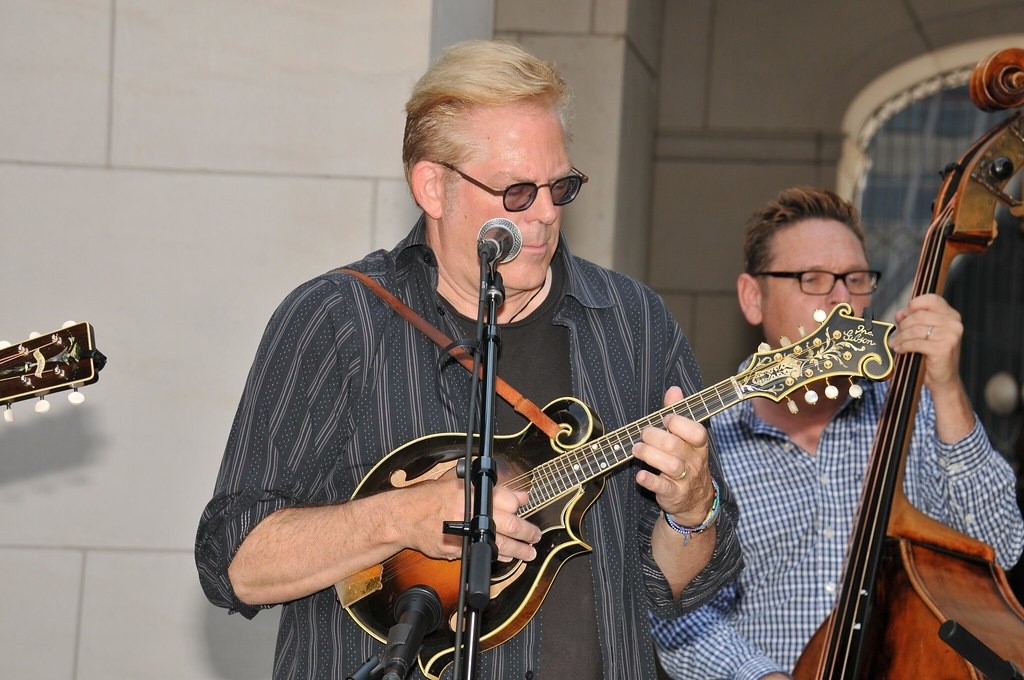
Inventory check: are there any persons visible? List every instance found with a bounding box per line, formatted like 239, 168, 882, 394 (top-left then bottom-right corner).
648, 184, 1023, 679
195, 39, 745, 679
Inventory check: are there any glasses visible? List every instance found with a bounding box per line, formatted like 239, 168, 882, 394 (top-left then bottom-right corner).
752, 269, 882, 296
427, 160, 589, 214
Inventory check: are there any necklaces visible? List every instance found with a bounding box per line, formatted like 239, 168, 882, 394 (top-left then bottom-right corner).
504, 275, 549, 324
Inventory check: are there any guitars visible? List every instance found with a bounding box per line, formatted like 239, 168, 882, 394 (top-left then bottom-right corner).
0, 318, 108, 424
327, 297, 900, 680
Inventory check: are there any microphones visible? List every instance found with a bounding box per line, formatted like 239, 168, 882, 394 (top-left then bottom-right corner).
476, 219, 522, 268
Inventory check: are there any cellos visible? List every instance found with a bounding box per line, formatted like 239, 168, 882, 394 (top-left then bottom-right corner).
781, 45, 1024, 680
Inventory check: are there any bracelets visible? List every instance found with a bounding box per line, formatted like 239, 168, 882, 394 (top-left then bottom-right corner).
663, 476, 722, 535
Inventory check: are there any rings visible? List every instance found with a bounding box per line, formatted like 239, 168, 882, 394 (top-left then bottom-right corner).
925, 326, 933, 340
672, 460, 689, 480
